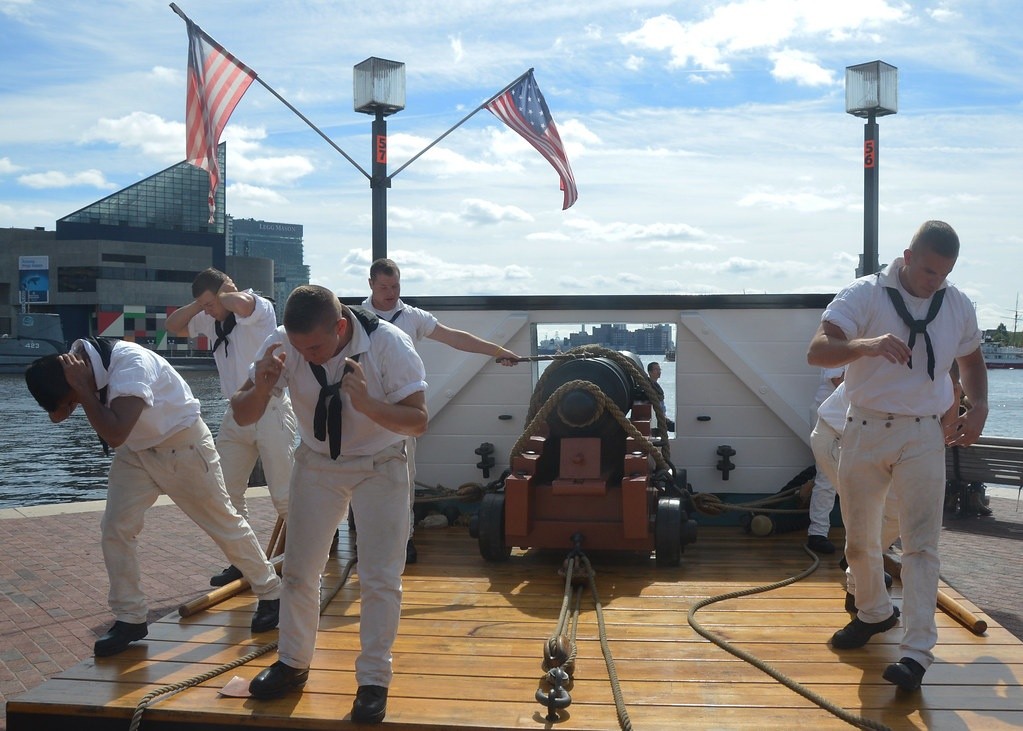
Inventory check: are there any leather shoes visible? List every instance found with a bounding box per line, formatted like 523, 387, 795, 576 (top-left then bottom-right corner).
831, 611, 897, 649
352, 684, 388, 725
248, 659, 309, 698
882, 661, 922, 690
250, 598, 280, 633
806, 533, 837, 554
94, 621, 148, 657
838, 554, 893, 588
405, 539, 417, 564
209, 563, 243, 586
844, 590, 900, 617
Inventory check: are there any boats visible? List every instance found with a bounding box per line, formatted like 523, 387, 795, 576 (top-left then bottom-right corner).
978, 334, 1023, 370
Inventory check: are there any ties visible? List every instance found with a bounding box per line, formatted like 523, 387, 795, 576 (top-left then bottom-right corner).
376, 309, 402, 324
875, 271, 947, 381
82, 335, 119, 456
308, 304, 379, 461
210, 295, 275, 358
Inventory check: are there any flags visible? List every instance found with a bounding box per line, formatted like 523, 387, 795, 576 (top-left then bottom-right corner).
186, 19, 258, 224
485, 73, 578, 211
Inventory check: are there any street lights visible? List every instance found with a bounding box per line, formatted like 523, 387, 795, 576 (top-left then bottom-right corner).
189, 340, 193, 356
147, 339, 155, 351
842, 59, 900, 278
168, 340, 175, 357
350, 54, 408, 263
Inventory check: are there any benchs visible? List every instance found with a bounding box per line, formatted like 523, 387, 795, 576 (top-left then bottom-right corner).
945, 435, 1023, 518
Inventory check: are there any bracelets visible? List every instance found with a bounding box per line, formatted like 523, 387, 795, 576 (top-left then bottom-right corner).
495, 346, 502, 355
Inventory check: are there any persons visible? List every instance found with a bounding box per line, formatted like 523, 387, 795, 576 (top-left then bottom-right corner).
164, 267, 298, 587
362, 259, 522, 367
807, 220, 991, 692
648, 362, 661, 381
230, 285, 428, 723
25, 336, 281, 657
